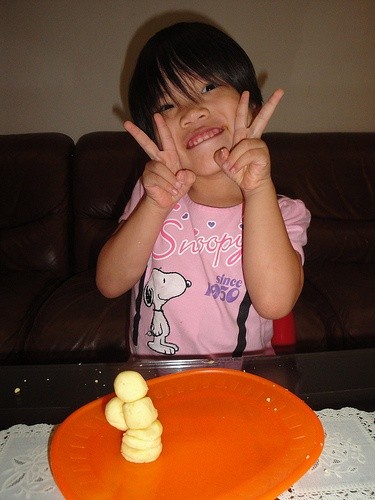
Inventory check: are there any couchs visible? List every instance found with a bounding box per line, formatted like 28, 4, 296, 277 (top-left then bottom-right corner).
0, 131, 375, 363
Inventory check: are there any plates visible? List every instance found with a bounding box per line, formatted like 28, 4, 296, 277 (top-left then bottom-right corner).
49, 369, 323, 500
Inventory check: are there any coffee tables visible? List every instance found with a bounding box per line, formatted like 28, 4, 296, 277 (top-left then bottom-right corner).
1, 352, 375, 499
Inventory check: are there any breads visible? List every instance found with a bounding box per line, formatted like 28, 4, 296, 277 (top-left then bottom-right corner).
105, 370, 163, 464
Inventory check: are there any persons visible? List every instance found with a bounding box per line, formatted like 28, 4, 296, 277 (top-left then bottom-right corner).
95, 19, 310, 362
